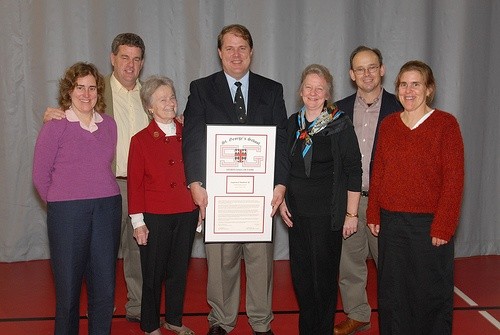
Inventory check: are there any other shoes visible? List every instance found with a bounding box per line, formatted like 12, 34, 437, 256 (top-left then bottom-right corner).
206, 323, 227, 335
165, 324, 194, 335
255, 329, 274, 335
124, 313, 141, 322
334, 316, 371, 334
145, 329, 161, 335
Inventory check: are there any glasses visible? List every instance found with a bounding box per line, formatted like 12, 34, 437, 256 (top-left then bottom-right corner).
350, 63, 381, 74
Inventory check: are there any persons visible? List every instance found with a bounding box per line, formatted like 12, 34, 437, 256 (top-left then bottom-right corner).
32, 63, 122, 335
182, 25, 292, 335
278, 64, 365, 335
44, 33, 184, 325
127, 76, 202, 335
365, 61, 464, 335
333, 46, 403, 335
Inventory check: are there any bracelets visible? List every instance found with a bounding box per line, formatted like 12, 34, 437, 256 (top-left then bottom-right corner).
346, 213, 358, 217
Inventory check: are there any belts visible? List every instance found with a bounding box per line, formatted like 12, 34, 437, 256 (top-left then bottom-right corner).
361, 190, 368, 197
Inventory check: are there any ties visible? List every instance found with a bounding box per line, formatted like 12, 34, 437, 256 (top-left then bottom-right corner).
232, 81, 247, 121
